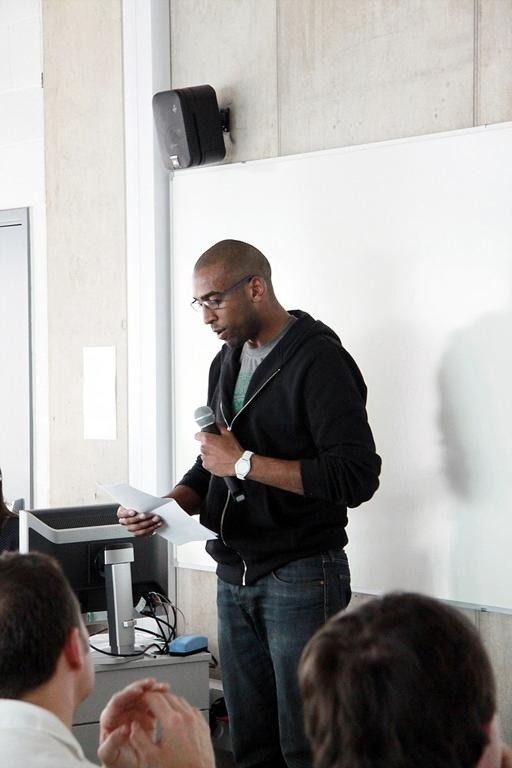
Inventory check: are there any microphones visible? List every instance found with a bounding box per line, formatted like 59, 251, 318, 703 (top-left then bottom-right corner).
194, 406, 242, 504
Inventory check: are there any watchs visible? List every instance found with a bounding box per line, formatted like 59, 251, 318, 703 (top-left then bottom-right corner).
233, 450, 255, 481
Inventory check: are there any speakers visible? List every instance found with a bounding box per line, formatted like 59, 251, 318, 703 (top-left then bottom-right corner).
152, 85, 229, 167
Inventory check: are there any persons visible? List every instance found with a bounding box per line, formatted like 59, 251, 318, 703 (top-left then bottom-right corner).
117, 240, 381, 768
0, 551, 215, 768
297, 592, 512, 768
0, 471, 19, 549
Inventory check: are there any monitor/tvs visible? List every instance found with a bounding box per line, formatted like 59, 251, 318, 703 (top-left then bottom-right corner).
19, 503, 176, 663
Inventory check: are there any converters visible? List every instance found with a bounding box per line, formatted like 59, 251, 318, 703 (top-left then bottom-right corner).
170, 636, 208, 657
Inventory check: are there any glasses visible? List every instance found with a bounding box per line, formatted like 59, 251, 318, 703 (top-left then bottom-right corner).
190, 274, 256, 312
